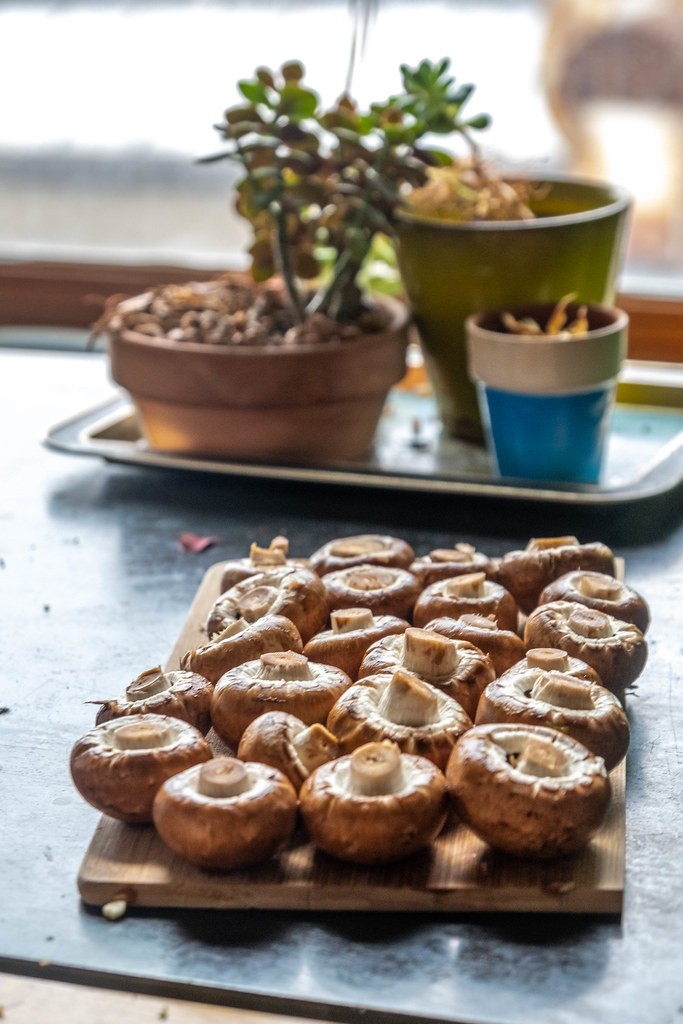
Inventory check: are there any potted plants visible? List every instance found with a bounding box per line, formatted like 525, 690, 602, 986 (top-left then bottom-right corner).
392, 162, 638, 453
103, 55, 495, 465
463, 288, 630, 484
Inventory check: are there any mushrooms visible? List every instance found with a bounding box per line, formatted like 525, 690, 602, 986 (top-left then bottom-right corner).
70, 534, 650, 866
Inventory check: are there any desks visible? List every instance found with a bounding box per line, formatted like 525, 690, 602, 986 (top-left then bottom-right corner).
0, 342, 683, 1024
